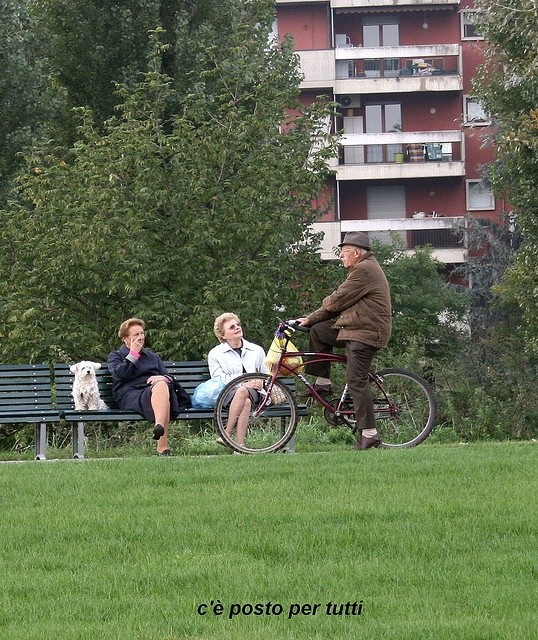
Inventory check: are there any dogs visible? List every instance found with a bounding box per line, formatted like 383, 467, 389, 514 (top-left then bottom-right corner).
68, 360, 109, 411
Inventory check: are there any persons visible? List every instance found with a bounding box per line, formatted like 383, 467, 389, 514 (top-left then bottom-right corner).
107, 318, 192, 453
295, 230, 391, 450
208, 312, 274, 454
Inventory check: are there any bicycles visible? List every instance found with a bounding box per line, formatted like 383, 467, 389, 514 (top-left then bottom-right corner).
212, 315, 438, 453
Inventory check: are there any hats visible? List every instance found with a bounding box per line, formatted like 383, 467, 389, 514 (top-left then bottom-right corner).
338, 231, 372, 251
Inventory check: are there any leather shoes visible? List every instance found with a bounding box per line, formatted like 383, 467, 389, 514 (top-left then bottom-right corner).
152, 424, 164, 440
296, 384, 333, 397
351, 433, 382, 451
156, 449, 174, 456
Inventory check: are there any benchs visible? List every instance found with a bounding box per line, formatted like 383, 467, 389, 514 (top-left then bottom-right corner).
54, 358, 309, 459
0, 363, 60, 460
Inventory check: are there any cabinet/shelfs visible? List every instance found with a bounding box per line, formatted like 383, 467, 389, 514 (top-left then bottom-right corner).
343, 116, 364, 165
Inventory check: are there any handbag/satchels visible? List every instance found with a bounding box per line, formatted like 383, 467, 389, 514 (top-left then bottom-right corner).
266, 384, 287, 407
172, 380, 191, 408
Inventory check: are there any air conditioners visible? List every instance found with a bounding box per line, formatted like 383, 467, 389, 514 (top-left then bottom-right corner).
338, 94, 361, 109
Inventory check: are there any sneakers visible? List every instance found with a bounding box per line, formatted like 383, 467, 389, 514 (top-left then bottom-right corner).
216, 437, 228, 447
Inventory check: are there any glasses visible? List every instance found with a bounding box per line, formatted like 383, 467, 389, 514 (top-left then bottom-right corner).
229, 323, 239, 330
